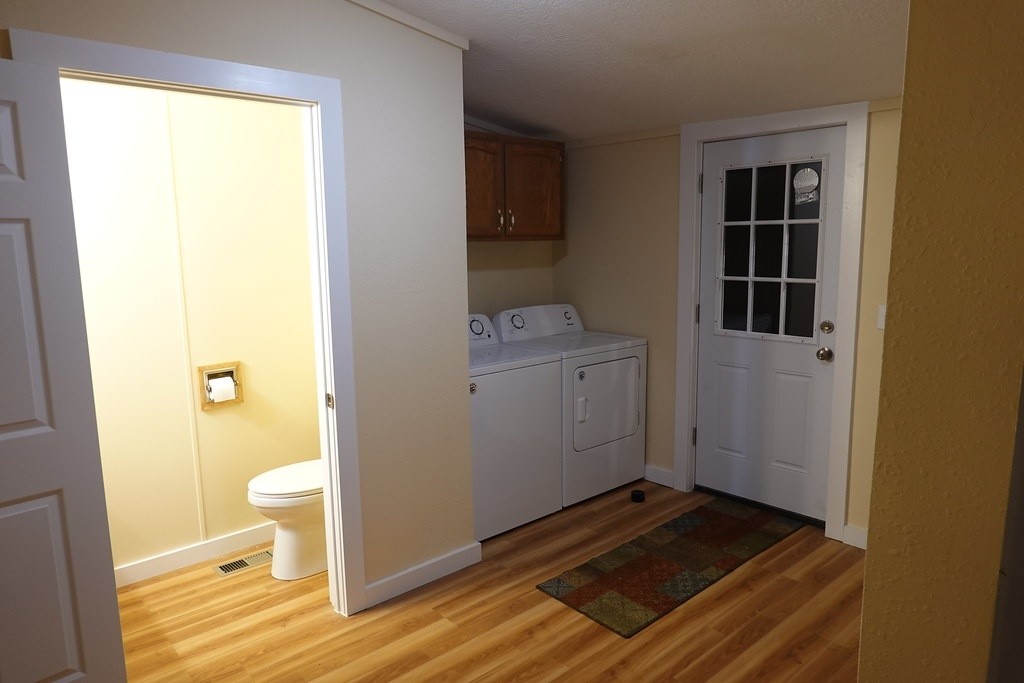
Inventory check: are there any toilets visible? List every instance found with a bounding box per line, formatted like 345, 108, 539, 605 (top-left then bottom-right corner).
247, 458, 329, 581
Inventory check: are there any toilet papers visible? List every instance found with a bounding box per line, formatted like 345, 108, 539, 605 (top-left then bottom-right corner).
208, 376, 236, 404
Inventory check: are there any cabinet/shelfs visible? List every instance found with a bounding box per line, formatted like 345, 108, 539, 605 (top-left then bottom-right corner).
464, 130, 567, 241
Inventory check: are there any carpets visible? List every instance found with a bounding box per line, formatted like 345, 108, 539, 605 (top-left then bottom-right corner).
535, 496, 806, 639
